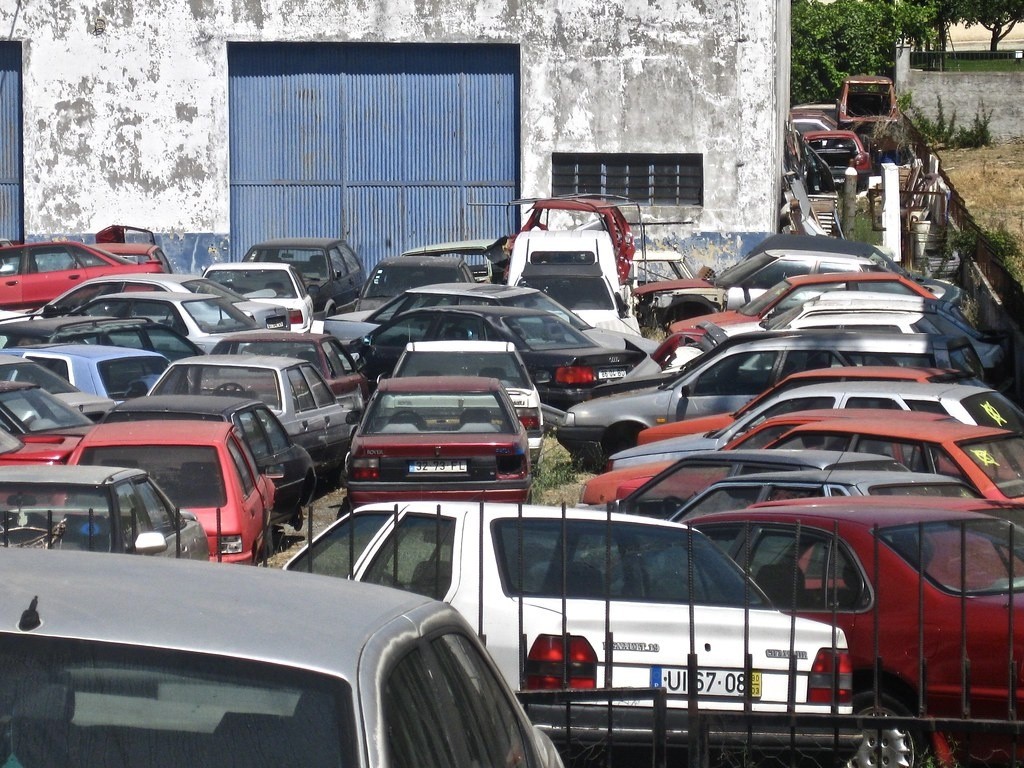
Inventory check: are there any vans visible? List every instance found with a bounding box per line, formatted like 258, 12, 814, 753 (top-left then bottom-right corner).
836, 76, 903, 134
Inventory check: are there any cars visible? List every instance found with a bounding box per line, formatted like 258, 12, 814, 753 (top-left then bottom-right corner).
0, 191, 1024, 768
787, 101, 873, 190
